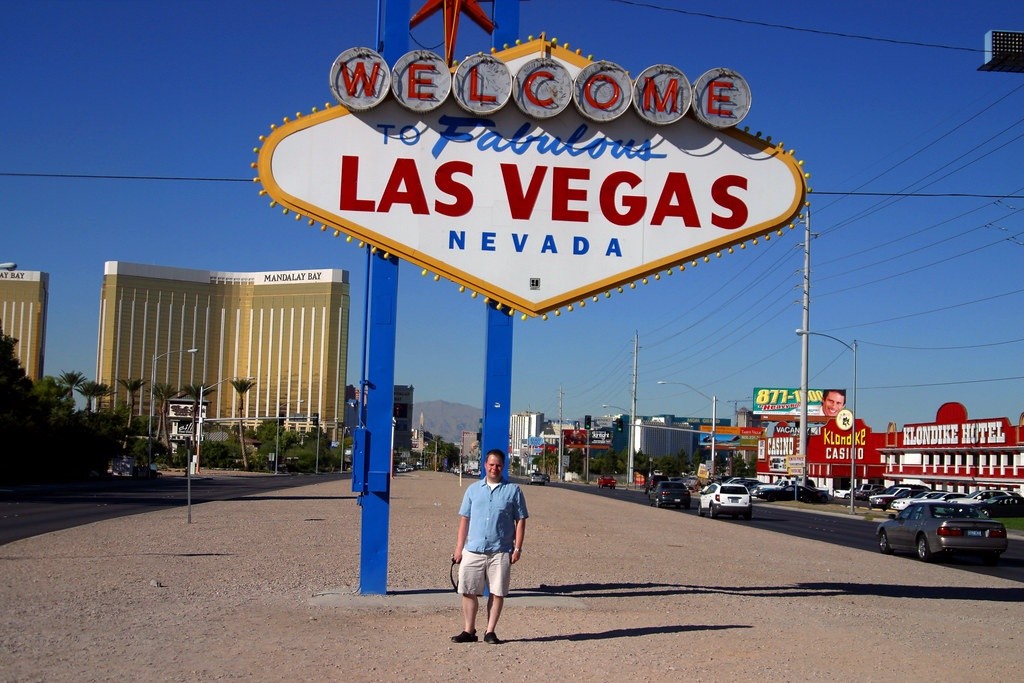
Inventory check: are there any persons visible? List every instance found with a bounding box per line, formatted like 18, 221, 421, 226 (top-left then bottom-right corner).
135, 464, 153, 480
819, 389, 846, 416
451, 449, 529, 644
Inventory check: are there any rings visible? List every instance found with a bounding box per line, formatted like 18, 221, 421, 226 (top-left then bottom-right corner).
452, 557, 454, 559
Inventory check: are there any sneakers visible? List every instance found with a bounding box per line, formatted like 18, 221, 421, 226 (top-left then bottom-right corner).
484, 630, 499, 644
451, 629, 478, 643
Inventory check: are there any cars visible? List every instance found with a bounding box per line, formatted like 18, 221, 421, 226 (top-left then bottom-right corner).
526, 472, 544, 485
598, 475, 616, 489
543, 474, 550, 482
395, 464, 416, 473
453, 467, 479, 476
729, 475, 830, 503
833, 484, 1024, 521
649, 481, 691, 510
875, 502, 1008, 565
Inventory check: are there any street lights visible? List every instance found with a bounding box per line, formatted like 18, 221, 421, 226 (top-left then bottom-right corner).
795, 329, 857, 514
603, 404, 631, 483
197, 376, 255, 474
658, 380, 716, 477
275, 399, 305, 473
546, 427, 564, 481
149, 348, 198, 477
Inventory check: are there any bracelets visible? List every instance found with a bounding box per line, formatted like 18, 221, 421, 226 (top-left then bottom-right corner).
514, 548, 522, 552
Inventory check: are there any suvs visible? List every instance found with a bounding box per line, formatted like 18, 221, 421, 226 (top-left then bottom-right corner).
697, 481, 752, 520
645, 472, 669, 494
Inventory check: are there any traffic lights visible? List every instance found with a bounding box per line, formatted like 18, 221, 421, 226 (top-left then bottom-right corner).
617, 418, 623, 432
585, 415, 591, 429
